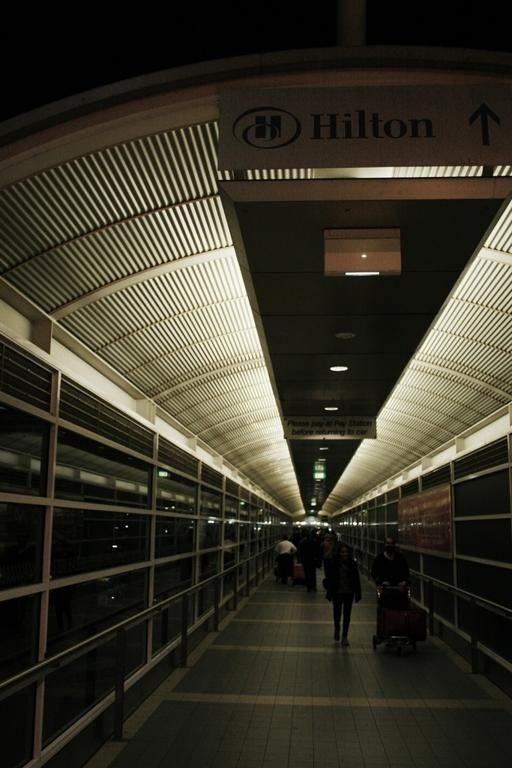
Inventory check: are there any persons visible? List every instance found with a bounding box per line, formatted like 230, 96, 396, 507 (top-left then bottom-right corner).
272, 524, 340, 593
328, 542, 360, 646
370, 535, 410, 594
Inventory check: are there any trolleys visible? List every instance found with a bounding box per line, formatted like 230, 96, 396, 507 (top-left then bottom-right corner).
370, 582, 418, 657
